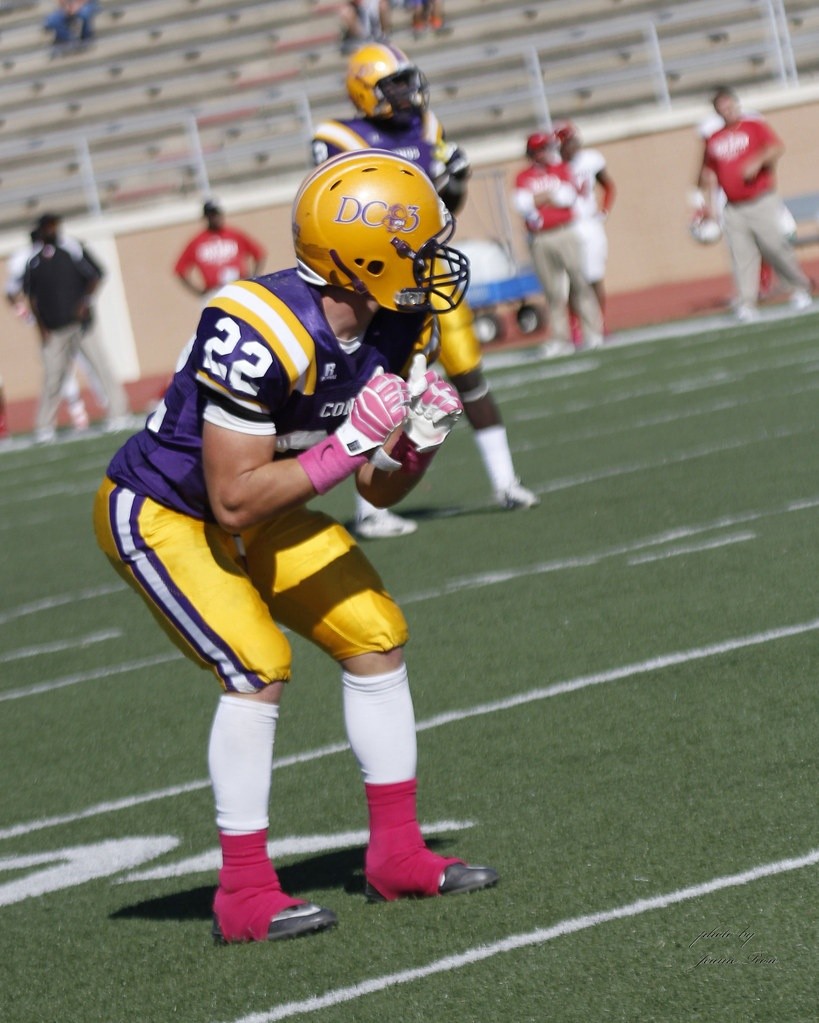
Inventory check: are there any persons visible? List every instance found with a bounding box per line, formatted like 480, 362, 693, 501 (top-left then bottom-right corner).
44, 0, 98, 44
514, 122, 617, 360
93, 149, 499, 947
696, 85, 813, 323
174, 200, 269, 297
5, 212, 128, 444
341, 0, 444, 45
311, 44, 538, 537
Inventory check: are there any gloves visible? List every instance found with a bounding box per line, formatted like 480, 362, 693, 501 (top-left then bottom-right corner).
386, 353, 463, 473
297, 363, 413, 498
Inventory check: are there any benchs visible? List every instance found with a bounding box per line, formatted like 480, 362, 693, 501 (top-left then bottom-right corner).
0, 0, 819, 239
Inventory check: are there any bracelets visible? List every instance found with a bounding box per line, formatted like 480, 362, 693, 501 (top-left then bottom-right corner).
390, 431, 433, 474
299, 434, 369, 496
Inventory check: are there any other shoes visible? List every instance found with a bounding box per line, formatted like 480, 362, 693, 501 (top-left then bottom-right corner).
366, 854, 500, 903
792, 288, 811, 308
495, 477, 539, 510
211, 899, 341, 946
580, 333, 602, 353
356, 506, 417, 538
734, 306, 756, 322
535, 339, 574, 357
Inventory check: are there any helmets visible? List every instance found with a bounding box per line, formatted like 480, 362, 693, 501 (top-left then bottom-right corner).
527, 133, 551, 153
292, 149, 470, 312
346, 41, 430, 133
693, 215, 722, 245
555, 123, 575, 140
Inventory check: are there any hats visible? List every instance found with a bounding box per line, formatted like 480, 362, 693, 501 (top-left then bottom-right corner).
200, 199, 223, 219
